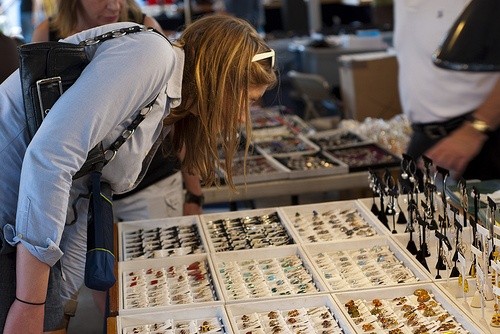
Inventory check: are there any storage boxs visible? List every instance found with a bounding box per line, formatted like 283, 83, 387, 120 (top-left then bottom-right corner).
336, 50, 402, 123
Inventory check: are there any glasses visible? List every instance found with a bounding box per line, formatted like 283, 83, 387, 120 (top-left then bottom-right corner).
251, 48, 276, 68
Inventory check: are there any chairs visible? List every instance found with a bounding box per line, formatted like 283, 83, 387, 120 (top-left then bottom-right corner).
288, 70, 340, 121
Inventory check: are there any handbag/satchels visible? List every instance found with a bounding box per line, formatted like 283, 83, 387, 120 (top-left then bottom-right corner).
16, 25, 174, 181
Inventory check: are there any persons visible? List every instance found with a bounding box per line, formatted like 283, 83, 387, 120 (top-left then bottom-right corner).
393, 0, 500, 194
32, 0, 162, 334
176, 0, 215, 32
0, 15, 277, 334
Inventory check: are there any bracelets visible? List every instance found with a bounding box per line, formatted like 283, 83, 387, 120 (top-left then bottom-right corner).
16, 297, 46, 305
466, 115, 494, 138
